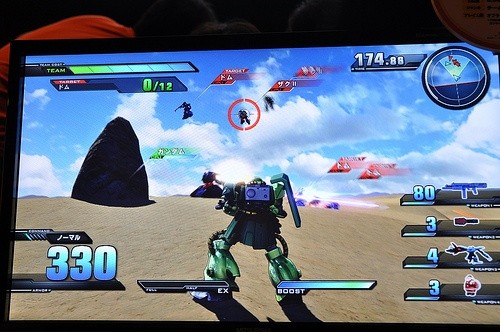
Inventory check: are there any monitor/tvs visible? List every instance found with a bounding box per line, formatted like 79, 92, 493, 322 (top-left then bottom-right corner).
1, 33, 500, 331
